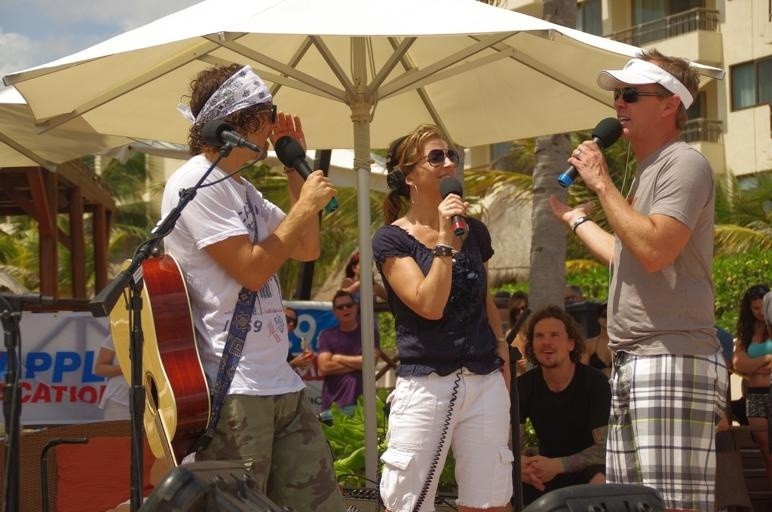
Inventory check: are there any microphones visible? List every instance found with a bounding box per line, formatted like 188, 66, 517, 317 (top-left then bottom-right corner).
274, 135, 339, 213
557, 117, 623, 189
202, 119, 263, 152
438, 175, 466, 237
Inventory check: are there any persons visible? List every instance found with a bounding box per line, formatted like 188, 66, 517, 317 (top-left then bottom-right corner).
550, 49, 730, 512
283, 253, 386, 423
162, 62, 349, 512
493, 284, 772, 499
372, 123, 515, 512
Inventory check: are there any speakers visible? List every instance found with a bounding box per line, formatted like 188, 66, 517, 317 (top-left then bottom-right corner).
138, 459, 291, 512
521, 483, 667, 512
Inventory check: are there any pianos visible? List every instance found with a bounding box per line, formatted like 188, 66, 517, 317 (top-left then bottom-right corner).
357, 302, 392, 315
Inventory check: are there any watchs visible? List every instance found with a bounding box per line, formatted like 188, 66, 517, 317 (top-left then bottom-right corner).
571, 216, 592, 235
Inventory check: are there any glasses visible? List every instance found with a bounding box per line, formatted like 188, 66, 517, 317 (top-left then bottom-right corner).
286, 315, 297, 324
335, 303, 353, 311
423, 150, 459, 167
614, 87, 659, 103
260, 105, 277, 123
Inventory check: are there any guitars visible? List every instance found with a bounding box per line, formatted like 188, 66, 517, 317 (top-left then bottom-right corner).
110, 255, 211, 470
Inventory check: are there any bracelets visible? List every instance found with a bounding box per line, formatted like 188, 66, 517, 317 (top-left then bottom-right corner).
432, 245, 453, 256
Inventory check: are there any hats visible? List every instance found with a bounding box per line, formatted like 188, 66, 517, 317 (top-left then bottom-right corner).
596, 58, 694, 110
